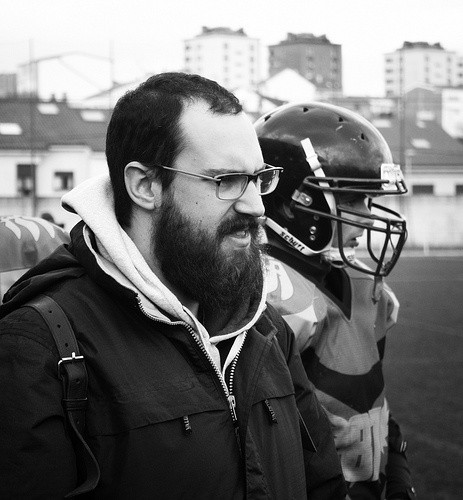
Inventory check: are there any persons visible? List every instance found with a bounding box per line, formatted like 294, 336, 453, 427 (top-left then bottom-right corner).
0, 72, 346, 500
253, 101, 418, 500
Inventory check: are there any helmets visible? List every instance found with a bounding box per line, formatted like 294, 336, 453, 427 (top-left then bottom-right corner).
252, 101, 408, 255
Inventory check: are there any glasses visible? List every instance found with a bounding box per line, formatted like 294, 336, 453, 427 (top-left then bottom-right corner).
141, 163, 284, 200
